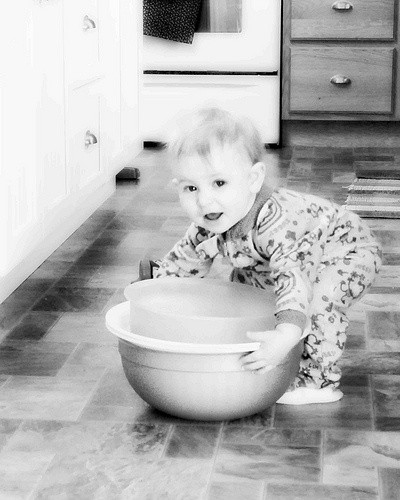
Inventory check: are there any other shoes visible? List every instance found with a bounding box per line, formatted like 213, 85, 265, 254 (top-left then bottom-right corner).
276, 387, 343, 405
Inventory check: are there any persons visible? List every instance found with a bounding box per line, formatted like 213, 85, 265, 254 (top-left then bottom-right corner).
155, 107, 383, 405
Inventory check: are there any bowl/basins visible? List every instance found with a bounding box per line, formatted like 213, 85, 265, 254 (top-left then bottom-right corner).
104, 277, 304, 422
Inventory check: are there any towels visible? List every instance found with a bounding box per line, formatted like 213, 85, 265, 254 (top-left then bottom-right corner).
143, 0, 202, 45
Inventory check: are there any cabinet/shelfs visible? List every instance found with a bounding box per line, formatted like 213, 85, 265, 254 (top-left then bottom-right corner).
0, 1, 279, 306
281, 0, 400, 145
